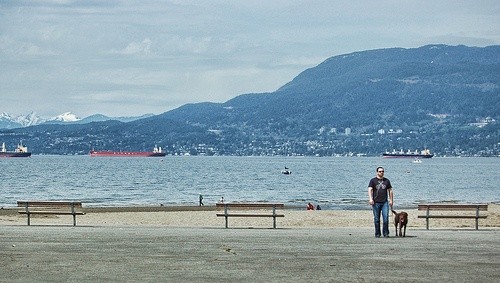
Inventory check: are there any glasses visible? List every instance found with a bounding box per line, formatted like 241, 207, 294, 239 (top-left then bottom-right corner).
378, 171, 384, 173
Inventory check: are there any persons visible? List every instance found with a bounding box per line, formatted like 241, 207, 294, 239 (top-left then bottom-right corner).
368, 167, 393, 237
307, 203, 313, 211
199, 193, 204, 206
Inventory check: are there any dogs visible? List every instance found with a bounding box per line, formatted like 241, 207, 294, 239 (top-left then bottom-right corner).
390, 208, 409, 238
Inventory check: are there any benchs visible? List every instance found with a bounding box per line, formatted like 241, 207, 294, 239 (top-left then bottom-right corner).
417, 204, 488, 230
216, 202, 285, 229
17, 201, 86, 227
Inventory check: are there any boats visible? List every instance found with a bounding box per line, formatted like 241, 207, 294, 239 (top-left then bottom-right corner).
0, 139, 32, 158
381, 148, 435, 159
88, 144, 167, 158
